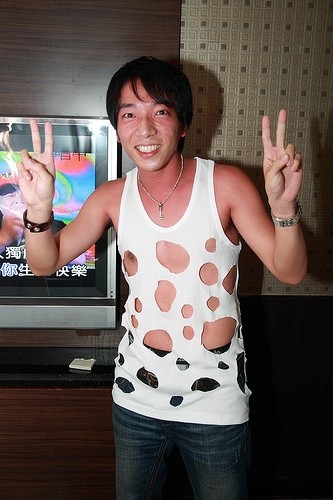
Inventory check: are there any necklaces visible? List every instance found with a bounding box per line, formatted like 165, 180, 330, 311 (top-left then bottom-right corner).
136, 153, 184, 219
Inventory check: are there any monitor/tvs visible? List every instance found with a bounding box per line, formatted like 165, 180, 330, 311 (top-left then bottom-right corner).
0, 115, 117, 330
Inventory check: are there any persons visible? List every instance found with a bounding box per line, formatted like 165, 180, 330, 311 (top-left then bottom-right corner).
17, 54, 311, 500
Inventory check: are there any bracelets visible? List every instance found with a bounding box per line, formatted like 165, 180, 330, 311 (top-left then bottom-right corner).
270, 203, 303, 228
